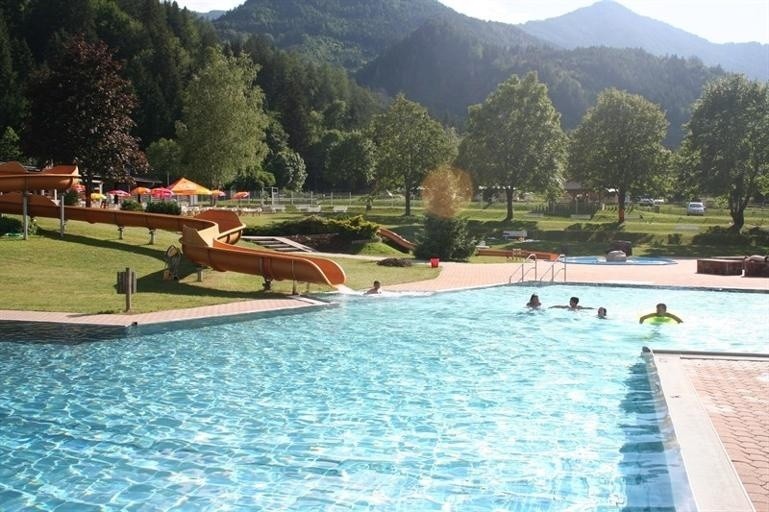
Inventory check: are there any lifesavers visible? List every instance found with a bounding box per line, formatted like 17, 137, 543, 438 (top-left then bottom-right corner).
640, 315, 679, 325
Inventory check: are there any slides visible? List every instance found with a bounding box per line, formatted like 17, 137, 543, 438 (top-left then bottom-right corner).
0, 161, 345, 287
378, 228, 558, 261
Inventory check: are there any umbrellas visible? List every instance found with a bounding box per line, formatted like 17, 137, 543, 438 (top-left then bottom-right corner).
72, 177, 250, 216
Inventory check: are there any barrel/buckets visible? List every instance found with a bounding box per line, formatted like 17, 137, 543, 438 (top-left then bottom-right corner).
430, 258, 440, 268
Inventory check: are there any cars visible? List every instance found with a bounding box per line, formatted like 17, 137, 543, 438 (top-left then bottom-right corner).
655, 199, 664, 207
639, 198, 654, 207
687, 201, 704, 216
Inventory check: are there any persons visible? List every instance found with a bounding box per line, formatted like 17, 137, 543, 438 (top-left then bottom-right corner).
597, 307, 607, 319
526, 294, 541, 309
548, 298, 595, 311
364, 281, 382, 294
639, 304, 683, 323
